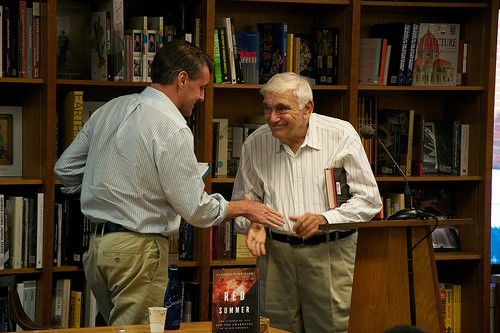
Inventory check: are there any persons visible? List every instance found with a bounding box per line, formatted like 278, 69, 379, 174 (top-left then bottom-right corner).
53, 40, 285, 325
230, 72, 383, 333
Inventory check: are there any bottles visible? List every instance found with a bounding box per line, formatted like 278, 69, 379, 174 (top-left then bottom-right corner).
165, 263, 183, 330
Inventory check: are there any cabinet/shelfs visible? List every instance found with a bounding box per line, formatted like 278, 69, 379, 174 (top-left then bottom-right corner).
0, 0, 500, 333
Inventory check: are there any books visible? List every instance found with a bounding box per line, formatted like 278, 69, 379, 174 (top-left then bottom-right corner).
1, 1, 471, 333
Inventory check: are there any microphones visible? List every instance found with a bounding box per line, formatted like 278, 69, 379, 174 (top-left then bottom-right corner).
359, 125, 426, 221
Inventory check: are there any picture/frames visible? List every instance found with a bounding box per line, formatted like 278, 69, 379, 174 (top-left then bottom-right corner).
0, 106, 23, 176
411, 183, 461, 251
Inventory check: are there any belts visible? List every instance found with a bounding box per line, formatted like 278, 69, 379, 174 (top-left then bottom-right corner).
93, 221, 133, 234
266, 229, 356, 246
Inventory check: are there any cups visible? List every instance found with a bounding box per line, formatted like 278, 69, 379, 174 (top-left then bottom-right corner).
148, 306, 167, 333
